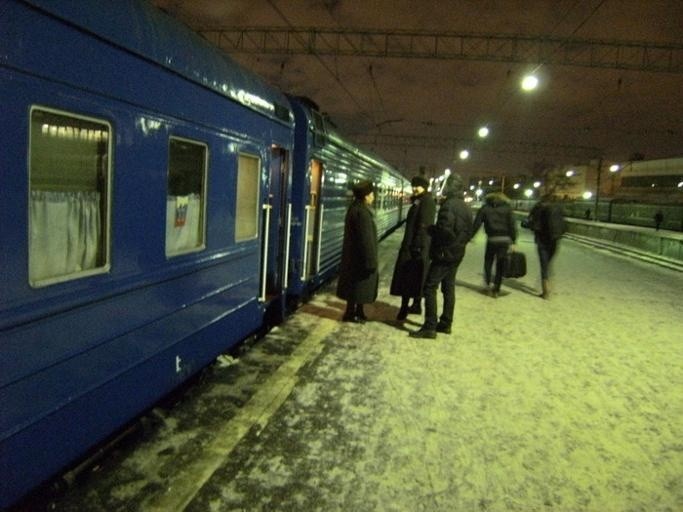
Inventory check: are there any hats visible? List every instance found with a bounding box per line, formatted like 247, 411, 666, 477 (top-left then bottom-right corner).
410, 175, 430, 186
352, 180, 373, 197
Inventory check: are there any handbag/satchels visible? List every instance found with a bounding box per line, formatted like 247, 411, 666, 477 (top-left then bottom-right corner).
502, 251, 527, 278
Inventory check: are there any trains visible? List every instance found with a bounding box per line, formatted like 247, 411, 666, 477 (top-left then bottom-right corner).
507, 198, 683, 232
0, 0, 414, 512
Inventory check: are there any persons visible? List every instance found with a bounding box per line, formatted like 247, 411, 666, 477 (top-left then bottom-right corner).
472, 191, 519, 297
336, 180, 379, 323
521, 194, 568, 299
409, 172, 472, 339
390, 176, 436, 321
651, 210, 665, 231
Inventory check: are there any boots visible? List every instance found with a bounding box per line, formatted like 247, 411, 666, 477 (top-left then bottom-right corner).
409, 324, 451, 339
479, 286, 500, 298
540, 278, 552, 300
396, 301, 422, 320
341, 311, 367, 322
